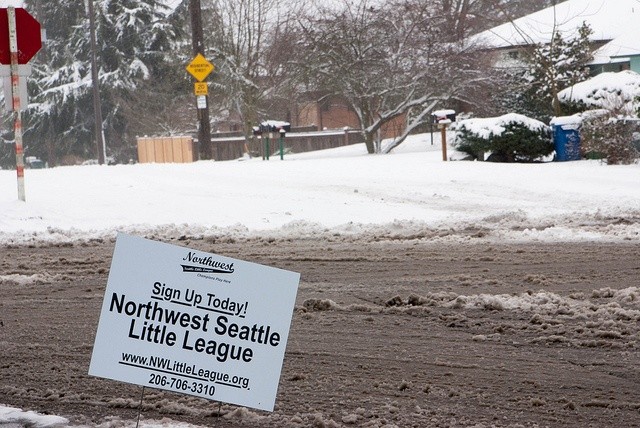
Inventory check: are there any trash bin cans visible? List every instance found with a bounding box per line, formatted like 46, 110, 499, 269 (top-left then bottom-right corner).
551, 117, 582, 161
